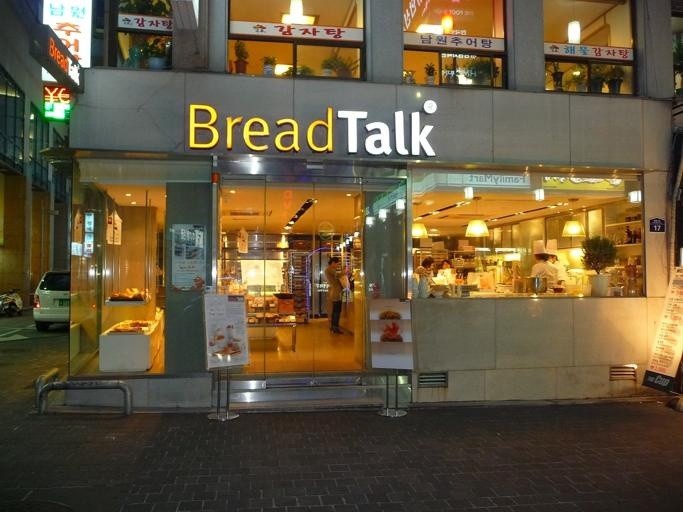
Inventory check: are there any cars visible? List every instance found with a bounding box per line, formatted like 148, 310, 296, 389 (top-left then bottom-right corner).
32, 269, 71, 334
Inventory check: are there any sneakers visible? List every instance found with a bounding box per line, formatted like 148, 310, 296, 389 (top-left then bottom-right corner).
329, 325, 342, 333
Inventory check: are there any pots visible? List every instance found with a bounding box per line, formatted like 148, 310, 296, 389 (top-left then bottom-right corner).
511, 276, 548, 295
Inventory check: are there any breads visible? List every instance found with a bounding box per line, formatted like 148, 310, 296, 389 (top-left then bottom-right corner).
110, 287, 145, 301
380, 310, 399, 319
114, 321, 149, 332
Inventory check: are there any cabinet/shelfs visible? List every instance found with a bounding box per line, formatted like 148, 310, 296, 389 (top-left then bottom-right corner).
603, 200, 644, 271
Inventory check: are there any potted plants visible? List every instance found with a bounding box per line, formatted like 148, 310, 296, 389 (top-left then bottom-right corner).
401, 55, 500, 86
129, 38, 169, 70
234, 39, 361, 78
545, 60, 625, 95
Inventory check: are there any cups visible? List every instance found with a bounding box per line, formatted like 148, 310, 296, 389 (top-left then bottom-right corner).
411, 277, 430, 298
232, 341, 245, 353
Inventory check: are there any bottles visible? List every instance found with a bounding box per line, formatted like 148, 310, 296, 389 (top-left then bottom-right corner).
612, 255, 643, 297
225, 324, 235, 348
612, 206, 641, 247
455, 254, 477, 279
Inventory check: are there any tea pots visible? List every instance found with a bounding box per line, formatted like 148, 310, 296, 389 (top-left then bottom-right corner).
213, 329, 225, 345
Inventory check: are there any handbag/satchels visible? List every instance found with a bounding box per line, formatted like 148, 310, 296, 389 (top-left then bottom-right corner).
337, 275, 351, 290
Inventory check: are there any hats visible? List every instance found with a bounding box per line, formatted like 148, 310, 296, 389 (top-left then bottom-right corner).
545, 238, 557, 256
532, 240, 545, 255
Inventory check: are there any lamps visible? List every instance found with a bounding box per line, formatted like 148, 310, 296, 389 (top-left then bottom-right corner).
465, 197, 490, 238
412, 203, 428, 239
562, 198, 586, 236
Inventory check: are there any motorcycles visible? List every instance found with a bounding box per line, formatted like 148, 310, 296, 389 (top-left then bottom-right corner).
1, 287, 26, 318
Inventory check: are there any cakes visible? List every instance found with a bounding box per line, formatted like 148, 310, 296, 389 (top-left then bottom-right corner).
380, 321, 402, 342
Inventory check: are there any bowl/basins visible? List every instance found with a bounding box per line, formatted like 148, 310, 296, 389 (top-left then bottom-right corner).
552, 288, 564, 293
432, 290, 444, 297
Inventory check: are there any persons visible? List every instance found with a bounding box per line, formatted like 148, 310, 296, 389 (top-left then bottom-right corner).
442, 259, 451, 269
323, 256, 347, 335
530, 252, 559, 284
546, 253, 566, 280
413, 256, 435, 279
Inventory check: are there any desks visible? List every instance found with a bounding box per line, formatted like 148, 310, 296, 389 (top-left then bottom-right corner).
246, 322, 297, 352
